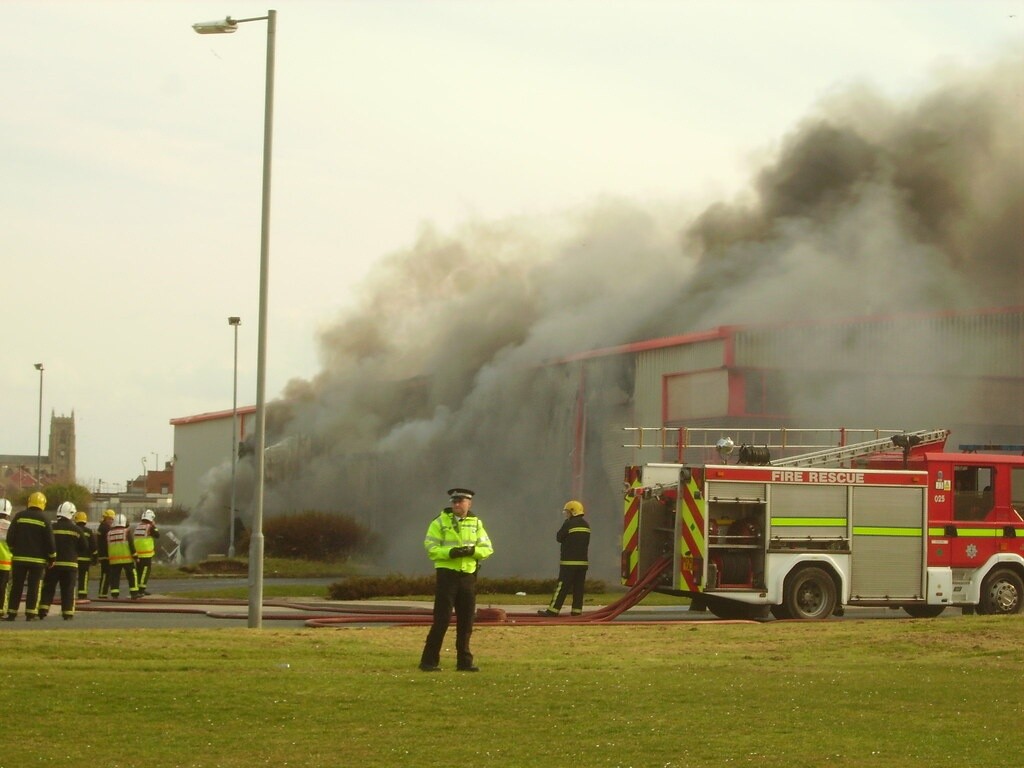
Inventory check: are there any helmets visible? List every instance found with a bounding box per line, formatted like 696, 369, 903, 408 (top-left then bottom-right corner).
113, 514, 127, 527
27, 492, 47, 512
75, 512, 88, 523
141, 509, 156, 523
103, 509, 115, 518
0, 499, 12, 516
564, 501, 584, 516
57, 501, 77, 520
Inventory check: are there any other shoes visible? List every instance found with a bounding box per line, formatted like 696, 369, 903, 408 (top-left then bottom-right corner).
457, 666, 480, 672
25, 615, 35, 621
0, 613, 16, 621
131, 593, 144, 599
537, 609, 558, 616
419, 664, 442, 672
140, 590, 151, 595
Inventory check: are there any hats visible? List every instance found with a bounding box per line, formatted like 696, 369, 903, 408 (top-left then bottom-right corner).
448, 489, 475, 501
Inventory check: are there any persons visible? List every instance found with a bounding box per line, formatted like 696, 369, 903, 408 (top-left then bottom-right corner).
0, 491, 160, 622
418, 488, 493, 672
537, 500, 591, 617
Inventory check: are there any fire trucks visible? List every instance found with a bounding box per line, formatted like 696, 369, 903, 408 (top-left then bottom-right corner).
617, 429, 1024, 621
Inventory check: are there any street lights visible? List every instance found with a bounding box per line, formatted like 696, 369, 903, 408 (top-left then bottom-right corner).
227, 315, 241, 557
33, 362, 45, 492
191, 9, 281, 628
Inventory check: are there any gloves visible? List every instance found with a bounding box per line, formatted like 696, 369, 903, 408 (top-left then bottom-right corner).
450, 546, 475, 558
